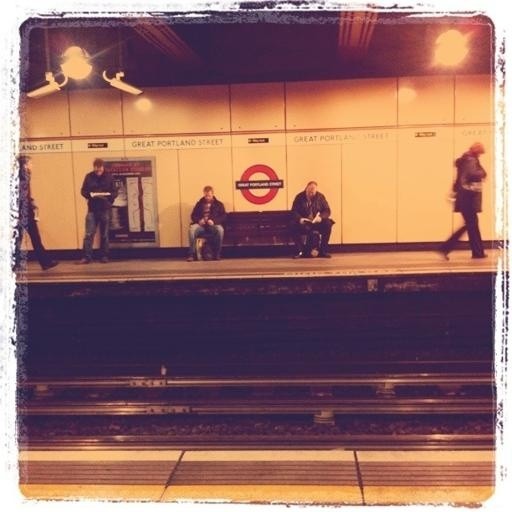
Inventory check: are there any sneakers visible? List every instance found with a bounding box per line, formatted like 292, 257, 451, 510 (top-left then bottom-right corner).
42, 262, 56, 270
186, 255, 197, 261
80, 259, 88, 263
15, 266, 25, 270
294, 252, 303, 258
101, 257, 108, 262
319, 254, 332, 258
473, 254, 487, 258
438, 246, 449, 259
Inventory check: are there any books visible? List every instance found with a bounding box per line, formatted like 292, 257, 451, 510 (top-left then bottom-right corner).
90, 191, 112, 199
303, 210, 320, 226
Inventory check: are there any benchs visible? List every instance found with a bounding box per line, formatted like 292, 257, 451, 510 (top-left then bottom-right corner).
203, 211, 308, 256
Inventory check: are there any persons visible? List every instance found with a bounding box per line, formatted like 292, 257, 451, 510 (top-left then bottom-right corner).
185, 186, 229, 261
436, 142, 490, 261
12, 155, 60, 271
288, 181, 336, 259
77, 157, 120, 267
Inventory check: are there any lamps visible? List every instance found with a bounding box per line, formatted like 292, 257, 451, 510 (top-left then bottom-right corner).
27, 44, 144, 99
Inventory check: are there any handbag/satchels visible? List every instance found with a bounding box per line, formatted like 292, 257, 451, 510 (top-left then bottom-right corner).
460, 172, 483, 192
305, 229, 320, 257
200, 243, 215, 260
